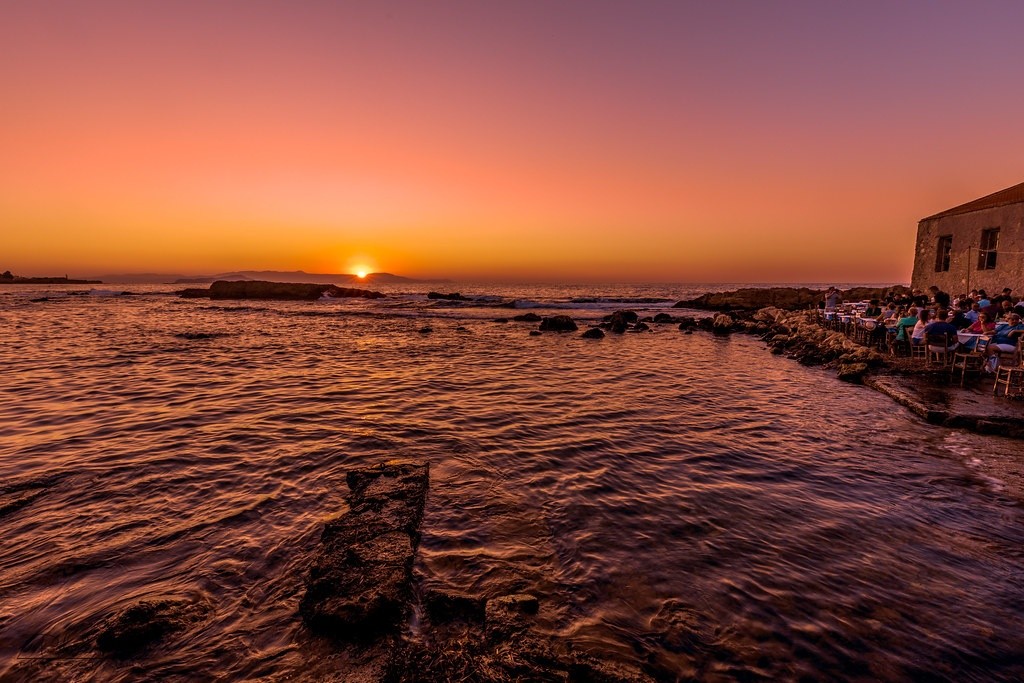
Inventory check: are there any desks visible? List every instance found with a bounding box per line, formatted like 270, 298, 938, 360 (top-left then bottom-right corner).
956, 330, 993, 345
824, 312, 836, 327
859, 316, 877, 343
838, 314, 854, 334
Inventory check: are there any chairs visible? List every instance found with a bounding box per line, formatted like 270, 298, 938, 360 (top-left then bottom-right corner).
808, 303, 1024, 395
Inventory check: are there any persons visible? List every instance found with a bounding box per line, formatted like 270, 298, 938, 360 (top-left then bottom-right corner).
865, 285, 1024, 374
824, 286, 842, 309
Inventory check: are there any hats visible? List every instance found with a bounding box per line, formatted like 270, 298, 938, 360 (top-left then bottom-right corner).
864, 321, 876, 331
